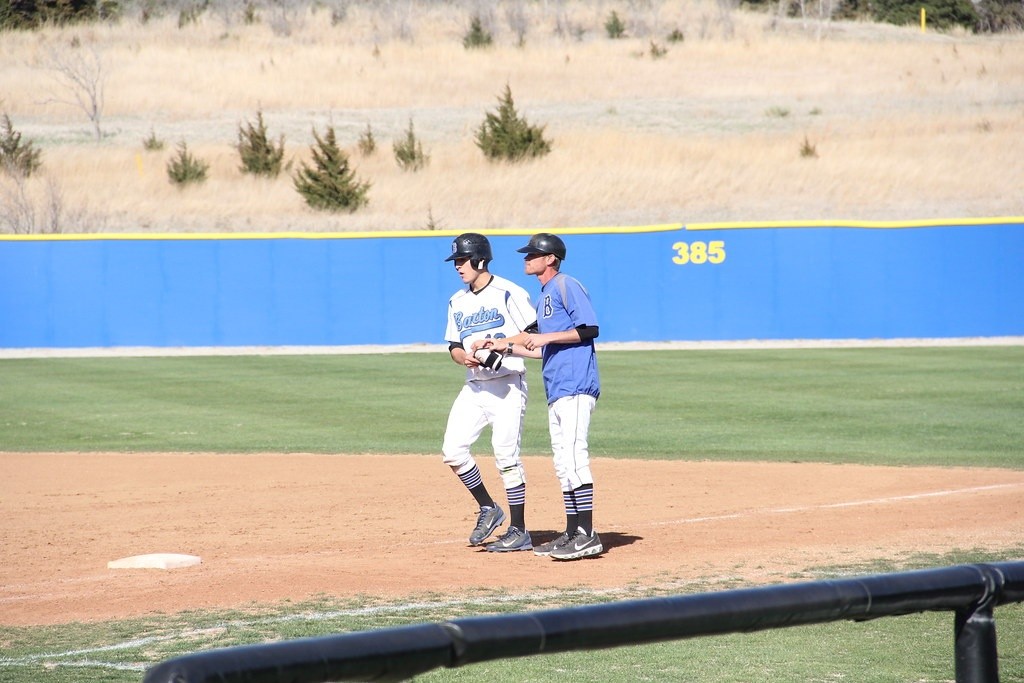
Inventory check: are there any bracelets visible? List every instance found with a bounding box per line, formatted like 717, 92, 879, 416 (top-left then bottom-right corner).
508, 343, 513, 355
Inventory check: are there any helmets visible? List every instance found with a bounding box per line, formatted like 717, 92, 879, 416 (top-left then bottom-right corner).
516, 233, 566, 260
444, 233, 492, 269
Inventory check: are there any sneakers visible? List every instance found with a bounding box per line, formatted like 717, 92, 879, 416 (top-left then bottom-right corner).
486, 526, 533, 551
548, 530, 603, 559
533, 531, 571, 556
470, 501, 506, 546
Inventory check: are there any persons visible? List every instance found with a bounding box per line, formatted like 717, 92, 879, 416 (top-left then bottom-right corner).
477, 233, 603, 559
440, 232, 539, 552
